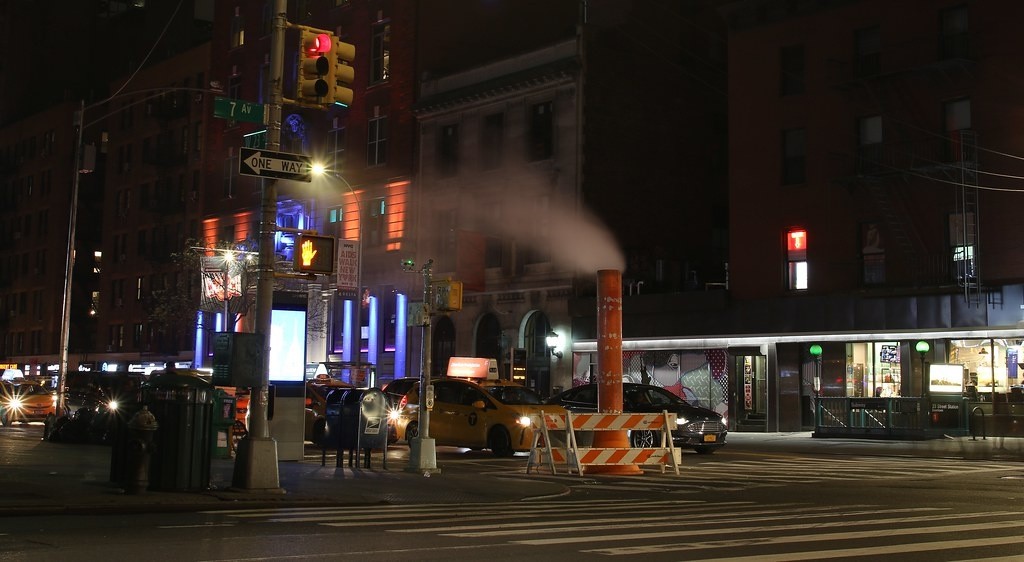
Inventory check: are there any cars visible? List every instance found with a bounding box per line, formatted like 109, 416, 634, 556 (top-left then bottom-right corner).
542, 383, 727, 454
64, 370, 147, 421
306, 374, 353, 449
0, 379, 68, 427
380, 378, 421, 407
393, 376, 573, 458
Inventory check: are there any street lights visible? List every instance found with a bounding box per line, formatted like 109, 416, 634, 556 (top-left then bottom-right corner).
916, 340, 931, 395
311, 165, 362, 367
810, 344, 823, 428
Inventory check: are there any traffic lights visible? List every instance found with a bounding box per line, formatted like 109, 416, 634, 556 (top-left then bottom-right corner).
296, 29, 333, 102
292, 233, 336, 277
332, 34, 356, 107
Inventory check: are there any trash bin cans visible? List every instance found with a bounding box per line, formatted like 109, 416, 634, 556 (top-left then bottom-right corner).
323, 386, 391, 470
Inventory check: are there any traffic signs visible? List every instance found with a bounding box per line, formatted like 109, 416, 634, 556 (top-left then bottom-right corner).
238, 146, 313, 186
